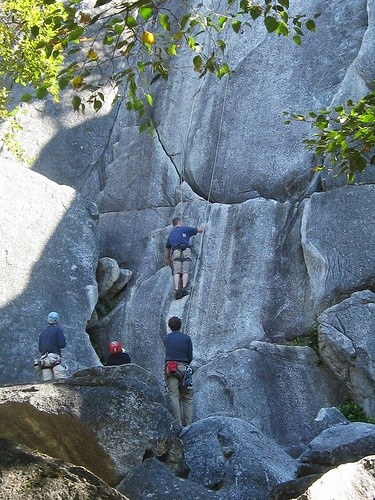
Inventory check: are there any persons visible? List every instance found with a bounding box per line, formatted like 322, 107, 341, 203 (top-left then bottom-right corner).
163, 316, 193, 427
106, 341, 131, 366
39, 312, 66, 380
165, 217, 203, 299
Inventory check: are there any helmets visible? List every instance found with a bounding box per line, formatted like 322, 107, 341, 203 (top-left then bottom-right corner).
109, 340, 122, 354
47, 312, 60, 324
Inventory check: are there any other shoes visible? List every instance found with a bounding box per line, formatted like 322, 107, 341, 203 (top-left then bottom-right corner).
182, 288, 188, 296
174, 290, 181, 300
